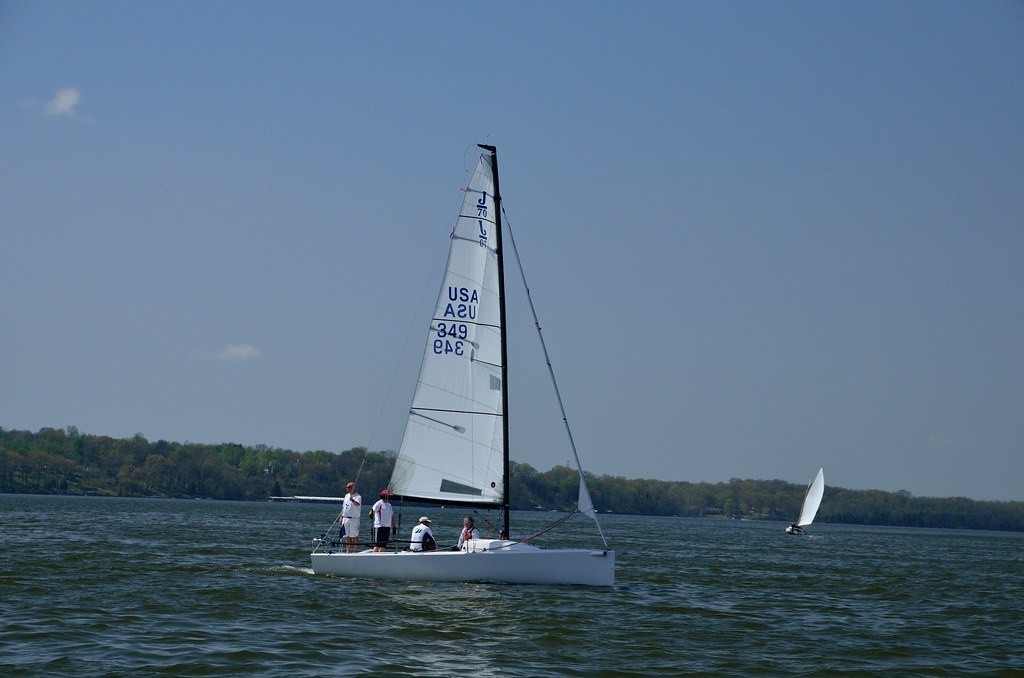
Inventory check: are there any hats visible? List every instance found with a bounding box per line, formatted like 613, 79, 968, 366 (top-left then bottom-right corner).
382, 489, 393, 495
420, 517, 432, 523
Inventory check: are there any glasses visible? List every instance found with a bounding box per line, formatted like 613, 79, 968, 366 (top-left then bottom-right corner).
346, 486, 352, 489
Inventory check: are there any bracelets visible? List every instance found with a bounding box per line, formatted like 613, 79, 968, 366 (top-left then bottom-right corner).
352, 499, 354, 502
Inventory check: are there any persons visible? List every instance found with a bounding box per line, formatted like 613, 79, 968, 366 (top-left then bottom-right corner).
369, 490, 396, 552
340, 483, 361, 553
451, 517, 480, 551
409, 516, 437, 552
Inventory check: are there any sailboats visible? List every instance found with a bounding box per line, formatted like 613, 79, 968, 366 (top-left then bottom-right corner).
785, 466, 824, 535
310, 144, 615, 584
